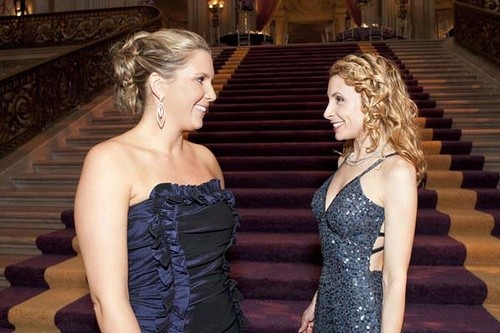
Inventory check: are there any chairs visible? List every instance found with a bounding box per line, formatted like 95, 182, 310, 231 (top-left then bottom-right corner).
325, 20, 412, 42
209, 24, 277, 47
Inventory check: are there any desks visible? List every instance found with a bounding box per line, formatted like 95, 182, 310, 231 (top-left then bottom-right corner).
337, 27, 396, 42
217, 33, 274, 46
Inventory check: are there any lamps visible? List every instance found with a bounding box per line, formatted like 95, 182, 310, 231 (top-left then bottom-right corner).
207, 0, 226, 15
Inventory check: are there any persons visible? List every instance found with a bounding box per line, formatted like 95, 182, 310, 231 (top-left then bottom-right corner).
297, 51, 429, 333
72, 28, 255, 333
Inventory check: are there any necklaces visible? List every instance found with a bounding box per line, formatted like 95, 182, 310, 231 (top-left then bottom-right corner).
344, 152, 378, 167
